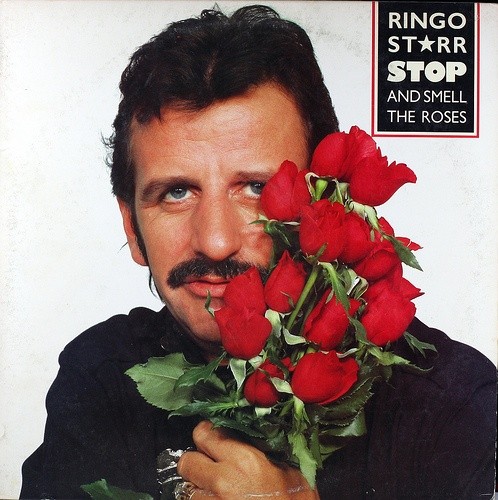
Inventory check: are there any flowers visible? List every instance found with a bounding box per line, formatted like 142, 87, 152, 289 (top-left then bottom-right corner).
79, 124, 438, 500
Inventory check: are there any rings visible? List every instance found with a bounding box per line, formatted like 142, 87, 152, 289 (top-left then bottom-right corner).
178, 485, 198, 500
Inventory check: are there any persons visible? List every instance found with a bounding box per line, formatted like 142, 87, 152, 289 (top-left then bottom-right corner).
19, 7, 498, 499
